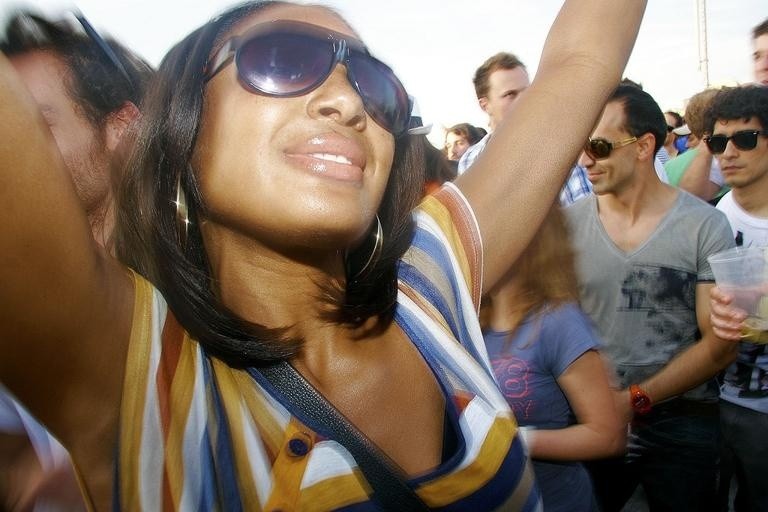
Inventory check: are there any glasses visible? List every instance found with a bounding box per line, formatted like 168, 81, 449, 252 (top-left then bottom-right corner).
704, 130, 767, 153
581, 137, 640, 160
0, 5, 136, 91
198, 19, 414, 136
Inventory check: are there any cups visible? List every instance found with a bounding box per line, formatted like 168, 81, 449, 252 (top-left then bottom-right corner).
705, 243, 768, 343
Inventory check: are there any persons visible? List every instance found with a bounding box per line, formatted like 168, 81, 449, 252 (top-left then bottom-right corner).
1, 0, 644, 512
425, 16, 768, 512
0, 7, 156, 512
